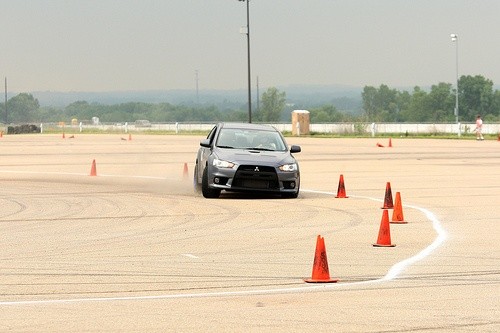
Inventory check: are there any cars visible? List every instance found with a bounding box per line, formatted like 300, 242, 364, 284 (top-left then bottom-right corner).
193, 123, 301, 198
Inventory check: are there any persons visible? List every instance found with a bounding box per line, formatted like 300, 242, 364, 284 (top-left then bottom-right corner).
476, 116, 483, 141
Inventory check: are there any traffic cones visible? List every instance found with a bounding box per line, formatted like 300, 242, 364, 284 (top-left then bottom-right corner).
381, 182, 395, 209
304, 235, 340, 283
182, 163, 190, 180
376, 137, 392, 147
88, 159, 98, 177
128, 133, 132, 140
389, 192, 408, 223
62, 132, 65, 138
372, 210, 396, 247
335, 174, 349, 198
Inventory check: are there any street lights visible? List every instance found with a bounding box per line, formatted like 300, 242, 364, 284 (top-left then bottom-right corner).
450, 33, 462, 138
